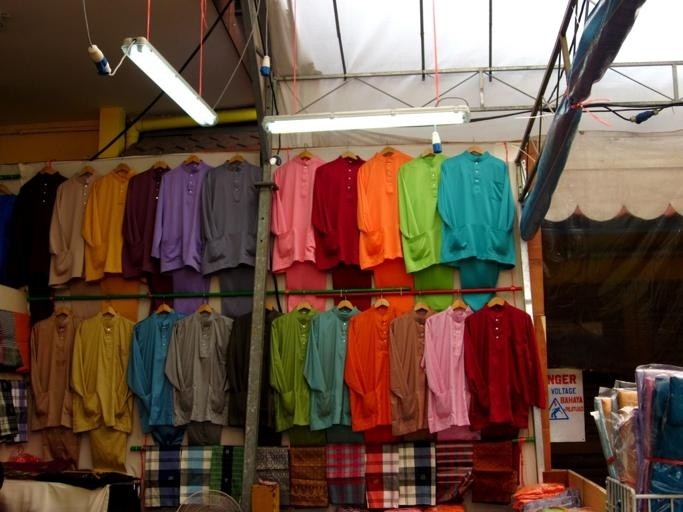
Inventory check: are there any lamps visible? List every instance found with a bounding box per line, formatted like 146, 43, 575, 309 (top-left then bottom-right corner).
133, 46, 218, 127
260, 105, 469, 134
